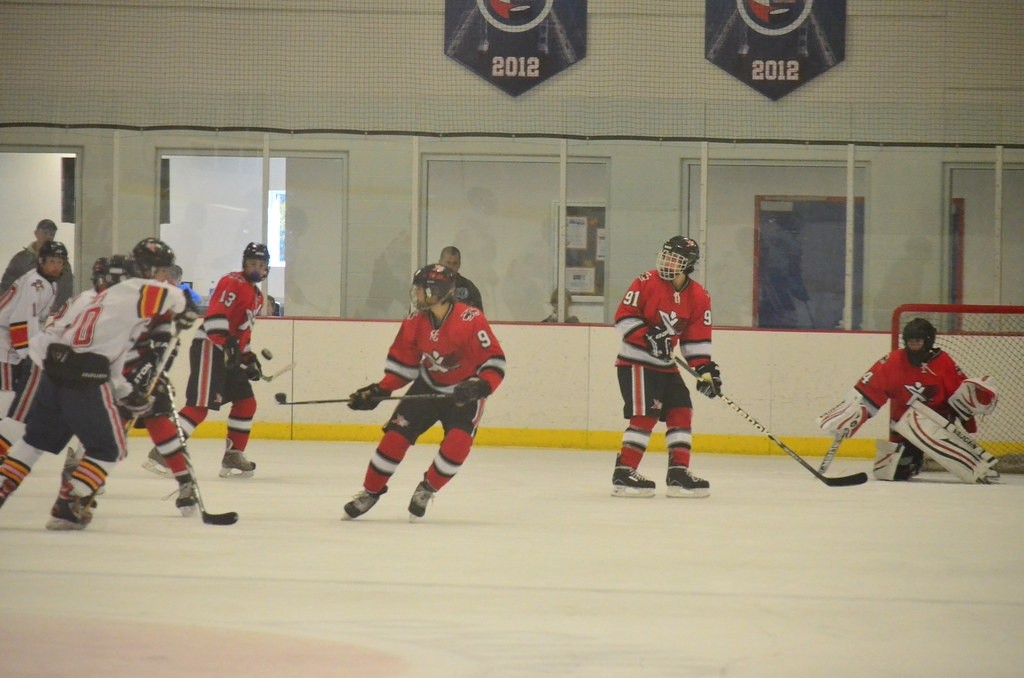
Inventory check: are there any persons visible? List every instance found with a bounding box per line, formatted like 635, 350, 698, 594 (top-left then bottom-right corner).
341, 263, 508, 523
0, 219, 73, 311
818, 318, 1000, 484
268, 295, 276, 315
0, 239, 198, 528
440, 246, 483, 310
542, 288, 579, 323
141, 241, 269, 477
611, 236, 722, 498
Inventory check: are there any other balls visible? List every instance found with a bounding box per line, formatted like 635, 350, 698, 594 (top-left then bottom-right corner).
261, 348, 273, 361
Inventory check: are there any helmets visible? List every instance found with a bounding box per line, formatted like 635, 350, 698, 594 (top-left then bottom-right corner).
902, 318, 936, 363
655, 236, 700, 280
92, 237, 175, 292
36, 240, 67, 282
408, 265, 455, 314
241, 243, 270, 282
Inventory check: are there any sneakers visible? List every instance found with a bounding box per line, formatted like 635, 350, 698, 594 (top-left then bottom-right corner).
176, 476, 199, 517
610, 463, 656, 498
343, 484, 388, 518
220, 451, 256, 479
406, 471, 433, 521
141, 446, 175, 478
666, 466, 710, 498
45, 497, 93, 529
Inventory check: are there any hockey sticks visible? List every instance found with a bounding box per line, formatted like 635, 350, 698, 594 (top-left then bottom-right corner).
166, 384, 239, 526
215, 343, 297, 382
668, 351, 868, 487
274, 391, 452, 405
84, 337, 178, 511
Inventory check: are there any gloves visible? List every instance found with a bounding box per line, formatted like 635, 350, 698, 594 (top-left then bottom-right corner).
223, 337, 242, 374
154, 333, 180, 372
454, 376, 490, 406
697, 361, 724, 399
349, 384, 391, 410
242, 352, 262, 381
128, 357, 170, 397
174, 290, 199, 330
122, 388, 155, 416
643, 324, 675, 362
